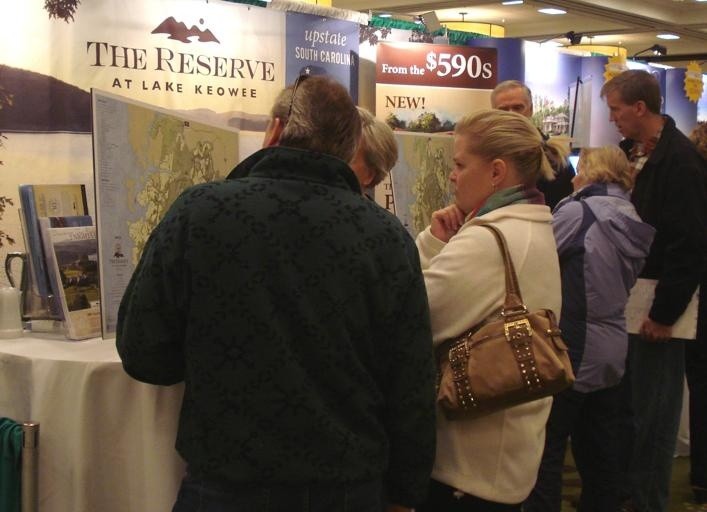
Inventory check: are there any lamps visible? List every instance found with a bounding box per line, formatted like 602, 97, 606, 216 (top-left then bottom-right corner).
632, 44, 662, 61
439, 20, 506, 38
556, 43, 628, 56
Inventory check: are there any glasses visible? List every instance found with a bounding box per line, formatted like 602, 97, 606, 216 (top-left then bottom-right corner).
285, 74, 311, 125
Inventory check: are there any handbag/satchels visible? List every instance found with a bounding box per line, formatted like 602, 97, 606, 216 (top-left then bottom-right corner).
436, 223, 576, 419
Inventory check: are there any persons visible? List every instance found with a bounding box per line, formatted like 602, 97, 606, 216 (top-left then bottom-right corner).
551, 143, 655, 512
414, 111, 563, 512
601, 70, 706, 512
116, 73, 436, 510
351, 104, 398, 201
492, 80, 576, 510
687, 123, 706, 505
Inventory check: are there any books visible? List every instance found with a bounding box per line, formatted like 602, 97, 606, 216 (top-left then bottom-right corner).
18, 185, 101, 340
